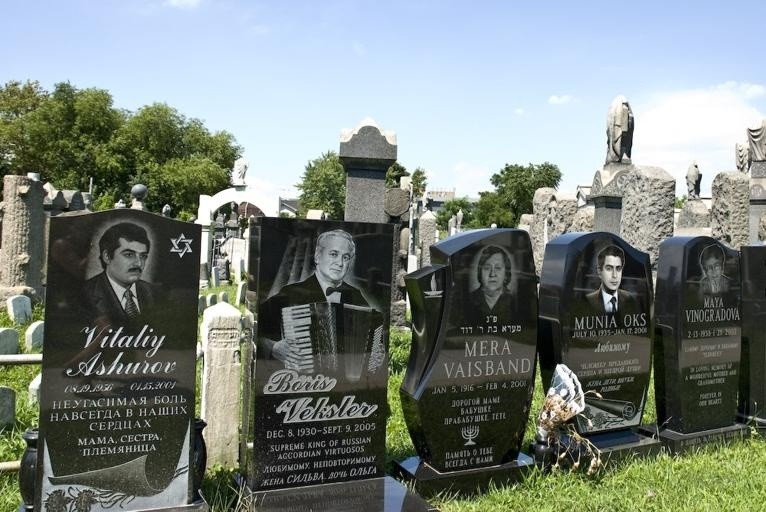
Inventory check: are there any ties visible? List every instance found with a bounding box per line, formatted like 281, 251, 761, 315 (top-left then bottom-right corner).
124, 292, 140, 321
611, 298, 617, 313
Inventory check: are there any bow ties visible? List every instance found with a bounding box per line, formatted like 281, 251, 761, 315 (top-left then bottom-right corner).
326, 286, 348, 297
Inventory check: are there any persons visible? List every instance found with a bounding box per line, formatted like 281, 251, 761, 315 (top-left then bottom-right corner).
691, 245, 739, 304
585, 244, 643, 315
82, 222, 155, 318
460, 245, 518, 324
259, 229, 386, 385
